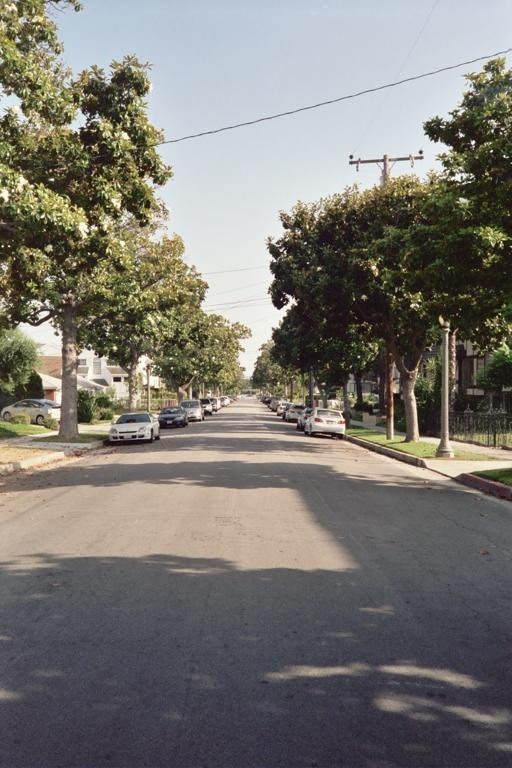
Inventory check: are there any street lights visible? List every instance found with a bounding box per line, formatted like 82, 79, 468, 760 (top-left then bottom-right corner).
145, 358, 152, 412
436, 315, 455, 458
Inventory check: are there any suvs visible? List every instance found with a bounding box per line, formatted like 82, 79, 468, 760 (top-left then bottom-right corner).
179, 399, 204, 422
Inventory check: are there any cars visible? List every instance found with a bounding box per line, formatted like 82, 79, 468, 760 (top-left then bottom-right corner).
1, 398, 61, 427
159, 406, 188, 428
108, 412, 160, 442
259, 393, 345, 439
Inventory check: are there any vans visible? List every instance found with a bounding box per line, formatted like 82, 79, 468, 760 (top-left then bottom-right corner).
200, 395, 231, 414
